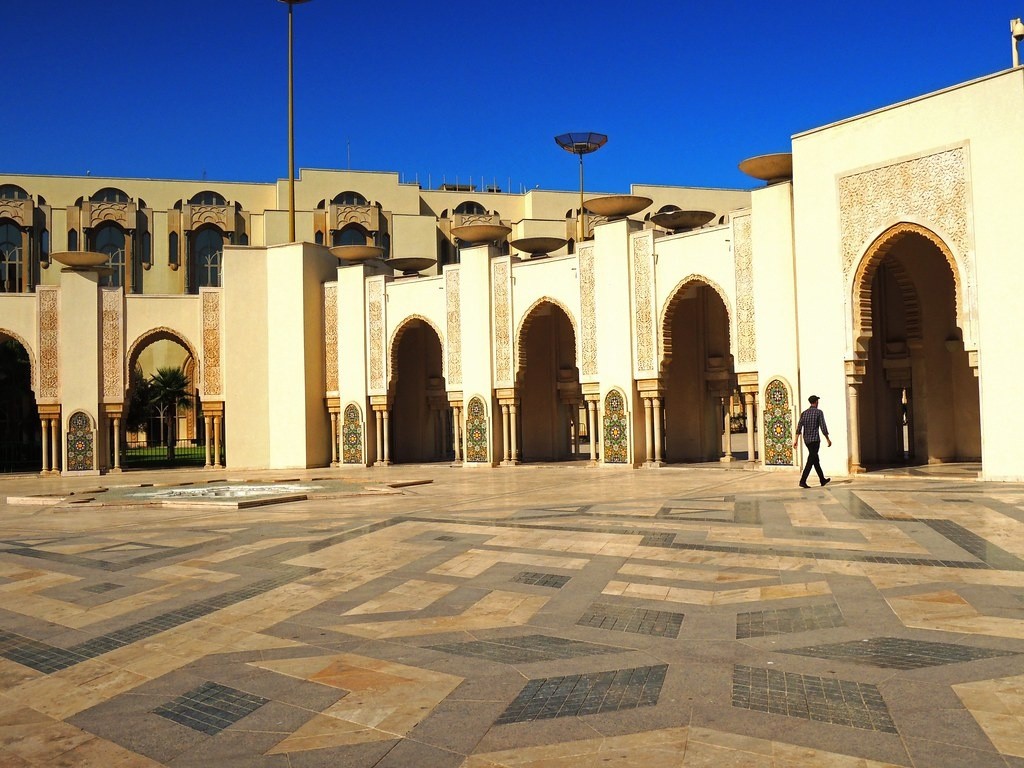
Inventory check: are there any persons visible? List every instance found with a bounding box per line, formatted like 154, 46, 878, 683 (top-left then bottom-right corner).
794, 396, 832, 488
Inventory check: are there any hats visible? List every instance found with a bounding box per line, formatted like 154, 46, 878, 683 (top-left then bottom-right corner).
808, 395, 820, 403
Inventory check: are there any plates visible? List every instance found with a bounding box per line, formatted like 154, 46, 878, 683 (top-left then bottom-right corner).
384, 258, 437, 275
50, 251, 115, 278
583, 196, 653, 220
450, 225, 512, 245
650, 210, 716, 233
510, 237, 568, 257
738, 153, 792, 185
329, 246, 385, 264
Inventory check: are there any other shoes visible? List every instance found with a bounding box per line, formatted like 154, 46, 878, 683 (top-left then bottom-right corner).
800, 481, 811, 488
821, 478, 830, 486
